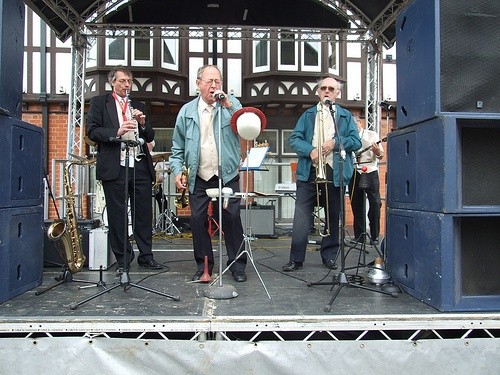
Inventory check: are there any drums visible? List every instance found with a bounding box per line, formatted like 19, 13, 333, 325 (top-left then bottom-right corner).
102, 195, 160, 240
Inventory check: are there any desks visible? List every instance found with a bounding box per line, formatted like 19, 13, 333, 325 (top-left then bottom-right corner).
208, 194, 281, 300
275, 189, 326, 224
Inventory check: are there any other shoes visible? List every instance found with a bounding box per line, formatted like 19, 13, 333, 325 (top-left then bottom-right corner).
323, 259, 338, 269
282, 262, 303, 271
193, 271, 212, 280
370, 237, 379, 245
233, 270, 247, 281
351, 238, 366, 244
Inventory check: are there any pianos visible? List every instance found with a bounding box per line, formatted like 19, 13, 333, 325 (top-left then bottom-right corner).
273, 181, 353, 238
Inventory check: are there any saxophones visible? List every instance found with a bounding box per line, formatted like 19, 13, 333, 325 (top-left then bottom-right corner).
46, 160, 86, 275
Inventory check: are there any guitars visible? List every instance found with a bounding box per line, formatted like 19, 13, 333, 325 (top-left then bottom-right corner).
352, 135, 388, 167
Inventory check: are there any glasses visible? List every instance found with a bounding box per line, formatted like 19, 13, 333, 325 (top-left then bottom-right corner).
320, 86, 334, 92
197, 77, 222, 86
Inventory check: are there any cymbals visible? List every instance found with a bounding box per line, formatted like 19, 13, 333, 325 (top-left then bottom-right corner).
67, 151, 97, 166
83, 134, 99, 149
150, 152, 173, 162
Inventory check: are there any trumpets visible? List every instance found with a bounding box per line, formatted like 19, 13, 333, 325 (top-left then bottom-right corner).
174, 167, 190, 210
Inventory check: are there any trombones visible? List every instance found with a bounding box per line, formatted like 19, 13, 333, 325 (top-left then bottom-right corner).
310, 102, 335, 238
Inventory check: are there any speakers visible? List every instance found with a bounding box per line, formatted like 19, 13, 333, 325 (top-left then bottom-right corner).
238, 205, 275, 236
385, 113, 500, 215
385, 207, 500, 311
395, 0, 500, 129
0, 0, 46, 306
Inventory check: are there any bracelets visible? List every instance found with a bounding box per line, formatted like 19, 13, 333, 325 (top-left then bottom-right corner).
375, 151, 383, 159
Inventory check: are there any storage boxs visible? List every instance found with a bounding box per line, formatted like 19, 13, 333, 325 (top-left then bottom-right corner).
88, 226, 117, 271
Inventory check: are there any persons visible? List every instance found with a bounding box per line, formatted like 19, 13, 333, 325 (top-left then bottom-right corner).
348, 115, 383, 247
282, 77, 361, 271
169, 64, 247, 282
86, 67, 168, 275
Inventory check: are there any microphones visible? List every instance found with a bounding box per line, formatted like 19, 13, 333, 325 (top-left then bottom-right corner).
131, 138, 145, 146
324, 97, 333, 105
377, 100, 394, 109
211, 93, 225, 99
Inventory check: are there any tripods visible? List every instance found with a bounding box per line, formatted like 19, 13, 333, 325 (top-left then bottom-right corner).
305, 105, 398, 311
32, 135, 181, 311
208, 147, 272, 301
344, 171, 403, 295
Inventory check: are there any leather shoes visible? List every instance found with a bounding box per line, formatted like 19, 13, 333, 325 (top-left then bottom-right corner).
115, 265, 129, 277
144, 259, 161, 268
